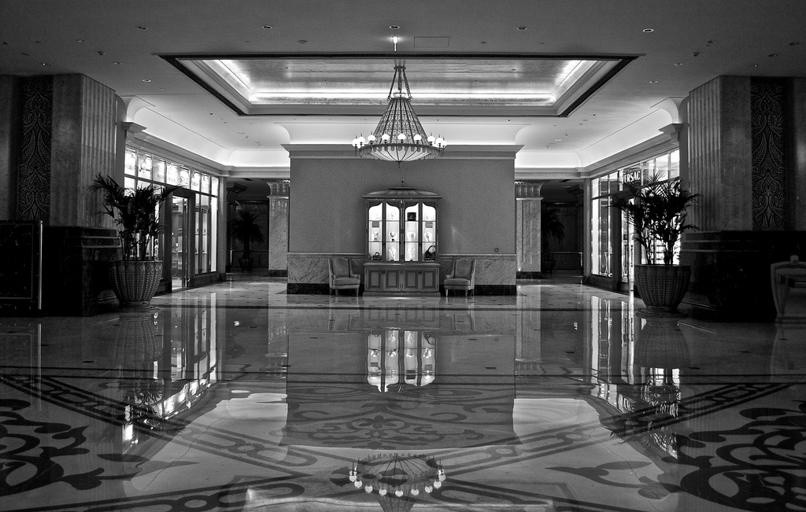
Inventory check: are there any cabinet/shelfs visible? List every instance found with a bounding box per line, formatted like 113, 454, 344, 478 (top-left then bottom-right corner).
360, 176, 442, 263
359, 297, 437, 394
402, 261, 441, 297
362, 261, 402, 296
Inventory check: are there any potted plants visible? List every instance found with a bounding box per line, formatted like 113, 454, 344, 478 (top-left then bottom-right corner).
83, 168, 187, 308
84, 313, 166, 447
516, 202, 566, 279
226, 208, 264, 272
601, 166, 703, 319
602, 320, 689, 456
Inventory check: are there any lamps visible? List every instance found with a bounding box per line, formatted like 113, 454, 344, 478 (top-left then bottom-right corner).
350, 56, 448, 168
349, 451, 445, 512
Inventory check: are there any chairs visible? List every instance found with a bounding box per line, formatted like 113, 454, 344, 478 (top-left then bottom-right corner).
443, 256, 476, 297
328, 297, 361, 333
327, 258, 360, 297
444, 298, 475, 333
349, 256, 362, 278
